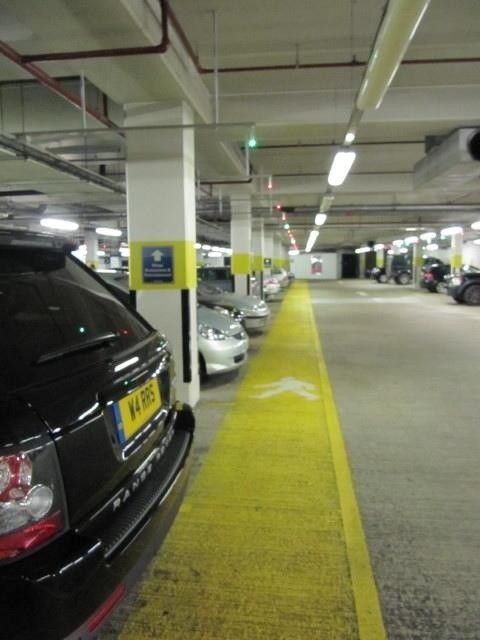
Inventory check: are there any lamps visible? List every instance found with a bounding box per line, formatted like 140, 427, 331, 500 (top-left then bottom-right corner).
355, 0, 433, 112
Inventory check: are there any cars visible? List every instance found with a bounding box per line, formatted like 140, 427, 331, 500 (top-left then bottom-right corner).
197, 253, 292, 300
365, 255, 480, 304
97, 269, 250, 380
196, 276, 271, 338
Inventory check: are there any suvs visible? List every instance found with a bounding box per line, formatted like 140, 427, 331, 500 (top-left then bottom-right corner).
0, 227, 196, 639
